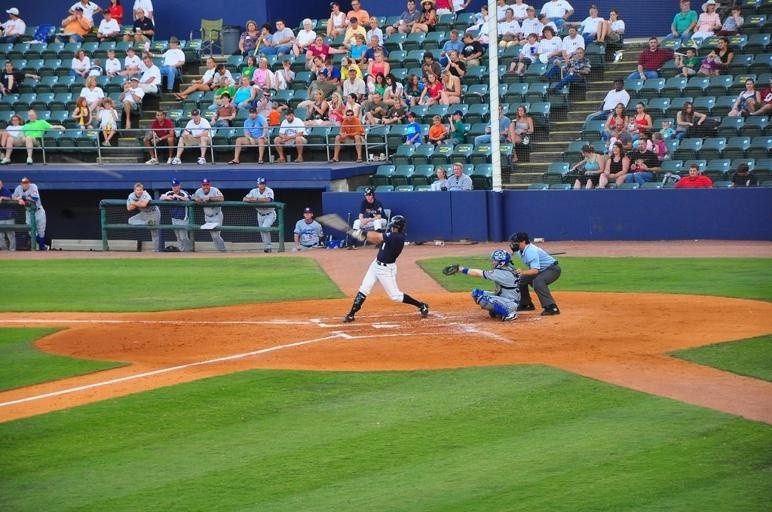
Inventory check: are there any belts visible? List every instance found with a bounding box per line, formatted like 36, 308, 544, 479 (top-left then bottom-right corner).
377, 262, 386, 266
206, 213, 217, 217
300, 243, 317, 248
259, 212, 271, 216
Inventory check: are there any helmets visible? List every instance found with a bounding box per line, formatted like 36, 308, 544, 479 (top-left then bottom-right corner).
489, 232, 529, 269
363, 187, 406, 229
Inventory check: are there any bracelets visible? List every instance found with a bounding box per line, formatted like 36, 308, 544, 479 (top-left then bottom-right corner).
462, 268, 469, 274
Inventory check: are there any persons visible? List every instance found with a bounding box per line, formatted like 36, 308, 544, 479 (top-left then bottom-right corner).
243, 176, 277, 253
127, 183, 161, 253
507, 231, 562, 316
192, 179, 226, 251
12, 176, 50, 251
293, 207, 324, 252
159, 179, 193, 252
2, 1, 186, 165
2, 183, 16, 251
572, 0, 771, 190
144, 0, 626, 192
343, 214, 430, 325
352, 187, 387, 233
458, 248, 522, 322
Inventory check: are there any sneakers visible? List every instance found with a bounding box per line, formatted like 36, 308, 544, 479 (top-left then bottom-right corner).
145, 157, 206, 165
27, 158, 33, 165
343, 314, 354, 323
420, 303, 428, 314
501, 302, 560, 320
264, 248, 272, 253
0, 158, 12, 165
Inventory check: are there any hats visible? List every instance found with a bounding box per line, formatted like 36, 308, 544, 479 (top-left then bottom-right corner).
701, 0, 721, 12
171, 177, 312, 213
5, 7, 19, 16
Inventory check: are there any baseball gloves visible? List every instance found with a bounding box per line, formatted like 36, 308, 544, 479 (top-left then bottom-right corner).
443, 266, 459, 276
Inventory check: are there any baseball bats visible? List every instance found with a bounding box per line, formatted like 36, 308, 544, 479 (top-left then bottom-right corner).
317, 215, 368, 242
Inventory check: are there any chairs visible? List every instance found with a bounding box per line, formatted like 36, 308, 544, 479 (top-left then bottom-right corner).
0, 0, 772, 192
363, 208, 391, 247
